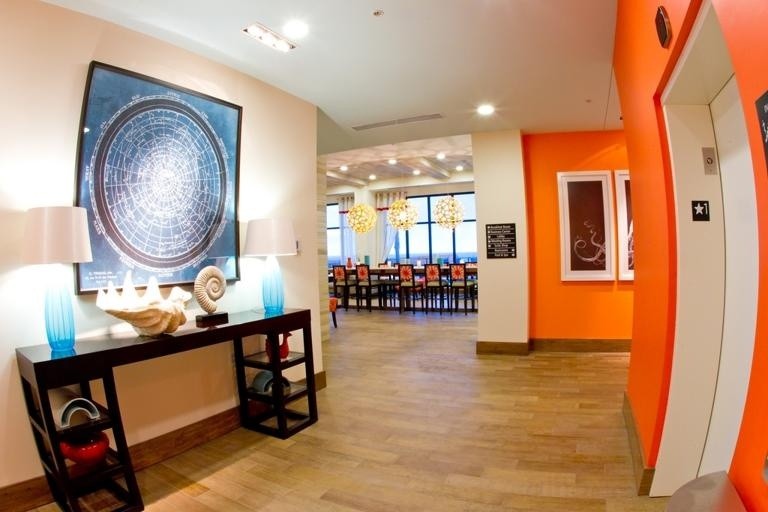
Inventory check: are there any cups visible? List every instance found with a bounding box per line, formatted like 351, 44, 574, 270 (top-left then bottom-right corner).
387, 260, 391, 268
416, 260, 421, 267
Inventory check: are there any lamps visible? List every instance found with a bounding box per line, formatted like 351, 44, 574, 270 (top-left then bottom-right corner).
433, 197, 463, 230
21, 206, 93, 354
387, 199, 419, 231
347, 202, 376, 233
243, 216, 298, 313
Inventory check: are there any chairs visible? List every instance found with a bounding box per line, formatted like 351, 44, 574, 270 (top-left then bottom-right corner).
331, 262, 475, 315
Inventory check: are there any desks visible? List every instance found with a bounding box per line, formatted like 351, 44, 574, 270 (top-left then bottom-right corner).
13, 302, 318, 512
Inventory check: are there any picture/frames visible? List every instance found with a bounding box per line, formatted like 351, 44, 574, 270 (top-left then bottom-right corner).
74, 60, 243, 295
556, 169, 618, 283
614, 169, 634, 282
